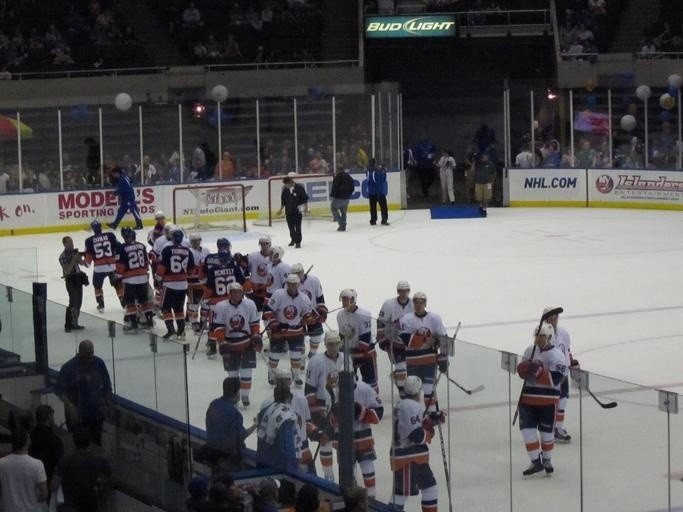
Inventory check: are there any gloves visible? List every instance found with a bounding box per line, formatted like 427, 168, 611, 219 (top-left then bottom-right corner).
427, 412, 445, 425
379, 338, 390, 351
421, 425, 435, 440
306, 422, 322, 441
304, 313, 316, 332
316, 303, 328, 322
354, 401, 365, 422
251, 334, 262, 353
438, 359, 449, 372
310, 408, 324, 426
393, 336, 405, 354
268, 319, 281, 333
219, 339, 231, 358
526, 360, 544, 377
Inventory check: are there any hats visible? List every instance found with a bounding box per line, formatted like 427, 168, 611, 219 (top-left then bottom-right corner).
188, 476, 209, 496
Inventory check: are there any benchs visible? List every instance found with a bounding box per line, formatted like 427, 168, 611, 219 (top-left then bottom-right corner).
364, 0, 555, 30
556, 0, 681, 58
507, 90, 681, 169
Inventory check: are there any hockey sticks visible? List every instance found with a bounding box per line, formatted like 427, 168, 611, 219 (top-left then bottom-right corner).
184, 323, 206, 360
230, 329, 276, 376
83, 195, 113, 232
437, 367, 485, 394
588, 390, 617, 408
308, 383, 335, 474
175, 292, 203, 341
512, 307, 563, 424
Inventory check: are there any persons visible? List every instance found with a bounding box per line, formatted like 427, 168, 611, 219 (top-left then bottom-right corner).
263, 274, 315, 382
137, 145, 238, 182
403, 142, 417, 199
541, 308, 579, 438
340, 119, 369, 172
260, 369, 317, 477
58, 236, 83, 332
554, 1, 681, 62
484, 140, 497, 183
187, 473, 370, 511
517, 322, 567, 474
330, 163, 354, 230
85, 219, 125, 311
0, 431, 47, 510
26, 403, 62, 508
436, 147, 455, 205
47, 421, 109, 510
464, 139, 479, 202
514, 133, 683, 169
131, 229, 154, 328
248, 238, 276, 319
243, 126, 329, 176
291, 265, 327, 360
201, 251, 242, 355
415, 126, 437, 197
54, 340, 113, 440
376, 283, 414, 366
277, 177, 309, 247
154, 230, 193, 338
367, 158, 388, 225
388, 376, 446, 512
337, 290, 377, 397
106, 167, 144, 229
264, 247, 291, 336
202, 238, 247, 272
149, 222, 188, 321
147, 215, 166, 246
114, 223, 152, 330
0, 0, 377, 81
188, 232, 209, 331
305, 332, 353, 482
393, 294, 445, 419
205, 377, 256, 470
256, 384, 301, 472
211, 282, 262, 404
0, 156, 113, 193
473, 153, 494, 216
326, 366, 383, 499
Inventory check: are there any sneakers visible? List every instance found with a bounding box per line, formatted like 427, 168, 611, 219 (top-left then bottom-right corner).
119, 296, 125, 306
162, 330, 176, 338
206, 340, 217, 356
65, 325, 70, 332
106, 223, 117, 229
292, 371, 302, 385
240, 390, 249, 406
201, 318, 208, 331
542, 459, 553, 473
308, 350, 316, 358
71, 325, 84, 329
337, 222, 346, 230
192, 323, 201, 332
554, 426, 571, 440
144, 318, 152, 326
122, 320, 137, 330
177, 332, 185, 337
96, 297, 105, 310
523, 460, 543, 475
133, 225, 143, 229
266, 370, 276, 385
295, 242, 300, 248
289, 240, 296, 246
324, 472, 334, 482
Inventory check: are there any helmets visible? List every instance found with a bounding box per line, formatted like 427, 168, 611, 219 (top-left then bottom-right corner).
91, 221, 101, 231
413, 294, 427, 308
291, 265, 305, 282
122, 227, 131, 237
536, 324, 554, 350
541, 308, 558, 327
172, 230, 183, 243
155, 210, 165, 219
339, 289, 358, 309
217, 239, 230, 251
164, 222, 177, 239
285, 274, 300, 295
269, 248, 282, 262
124, 231, 135, 244
325, 332, 340, 347
227, 282, 243, 296
403, 375, 422, 394
219, 250, 230, 263
259, 238, 271, 252
190, 233, 202, 245
398, 284, 410, 294
